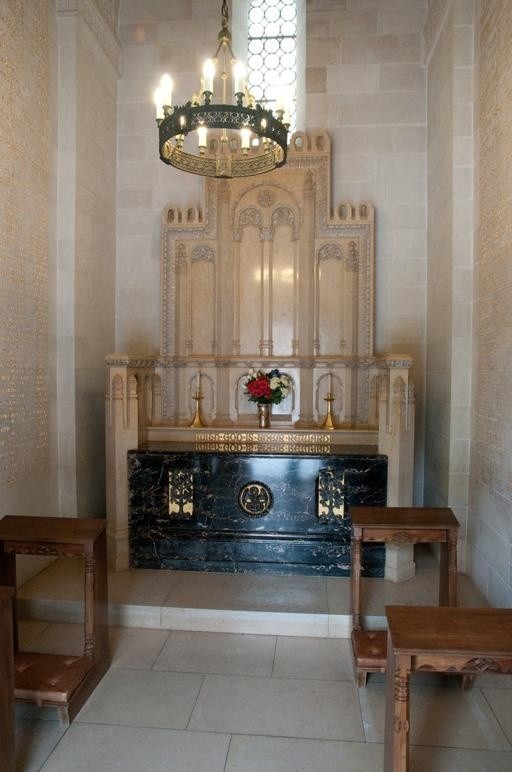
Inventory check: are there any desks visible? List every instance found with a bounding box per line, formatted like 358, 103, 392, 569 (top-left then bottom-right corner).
1, 586, 17, 771
0, 515, 108, 727
351, 505, 474, 692
383, 606, 512, 772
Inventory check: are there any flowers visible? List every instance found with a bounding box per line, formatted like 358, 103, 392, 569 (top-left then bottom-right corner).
240, 368, 291, 405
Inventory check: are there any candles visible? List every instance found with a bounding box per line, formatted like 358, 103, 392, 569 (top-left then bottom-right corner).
195, 372, 200, 392
327, 373, 331, 395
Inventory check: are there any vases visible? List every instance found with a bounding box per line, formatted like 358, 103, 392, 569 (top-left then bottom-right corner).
257, 404, 270, 426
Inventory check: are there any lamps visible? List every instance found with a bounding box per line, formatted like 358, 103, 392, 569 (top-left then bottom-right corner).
152, 1, 290, 177
